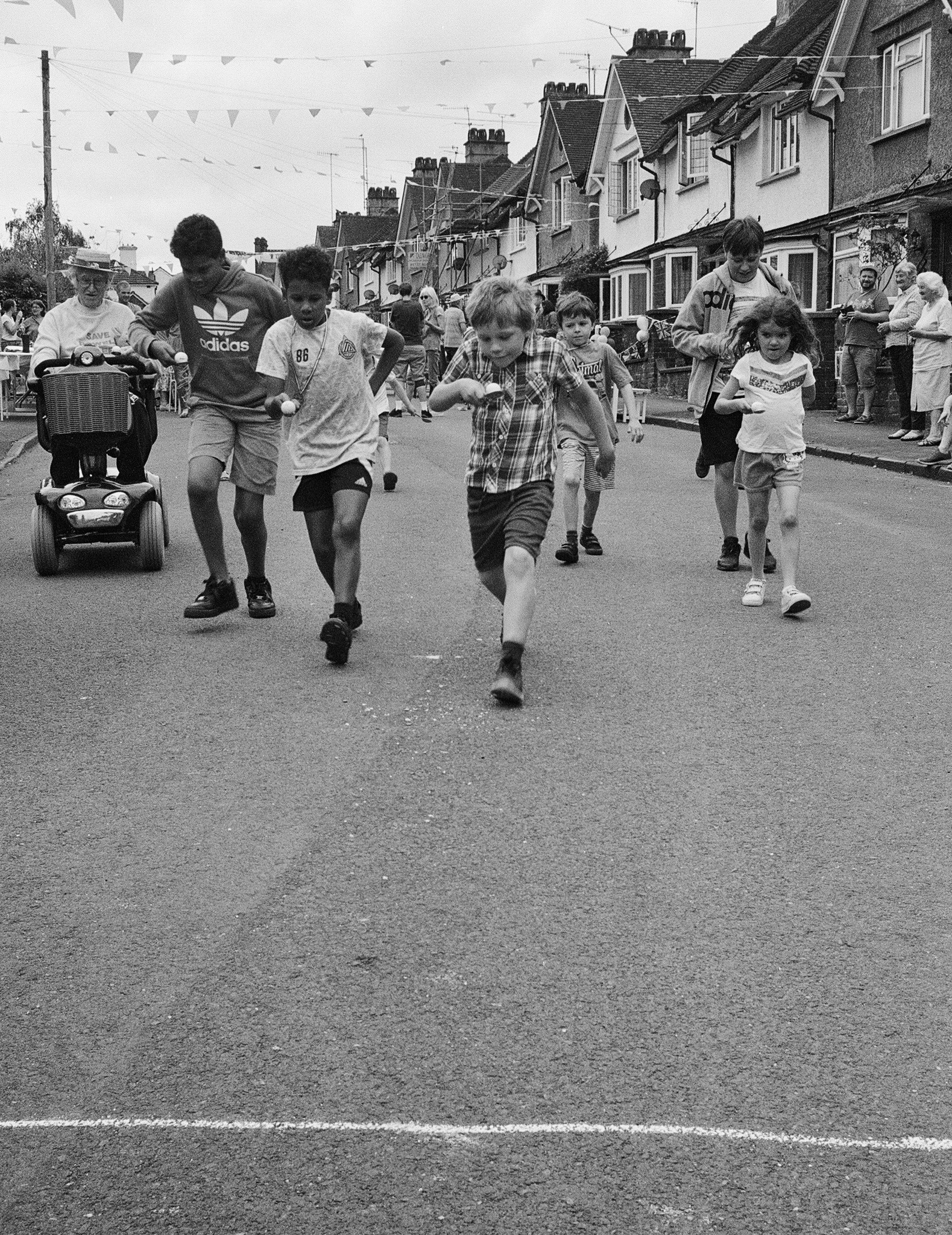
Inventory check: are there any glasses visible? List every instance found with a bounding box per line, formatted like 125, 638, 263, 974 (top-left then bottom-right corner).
75, 275, 108, 286
420, 296, 428, 299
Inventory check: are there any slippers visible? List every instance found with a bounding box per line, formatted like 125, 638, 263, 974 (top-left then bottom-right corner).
917, 437, 942, 447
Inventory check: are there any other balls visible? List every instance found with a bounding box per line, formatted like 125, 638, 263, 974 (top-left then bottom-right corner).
174, 352, 188, 364
751, 401, 763, 412
636, 316, 649, 342
281, 401, 296, 414
590, 326, 615, 349
486, 383, 501, 394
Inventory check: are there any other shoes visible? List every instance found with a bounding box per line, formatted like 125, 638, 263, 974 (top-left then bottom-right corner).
917, 448, 952, 469
743, 532, 777, 569
382, 471, 397, 491
888, 430, 910, 439
160, 404, 177, 411
421, 408, 432, 418
389, 409, 403, 417
490, 654, 526, 703
351, 597, 364, 629
834, 414, 858, 423
780, 587, 812, 614
716, 543, 741, 571
243, 576, 275, 618
180, 409, 187, 418
321, 614, 352, 664
900, 431, 924, 442
220, 471, 231, 481
578, 530, 603, 555
854, 415, 875, 424
184, 574, 240, 618
555, 541, 579, 562
742, 579, 767, 606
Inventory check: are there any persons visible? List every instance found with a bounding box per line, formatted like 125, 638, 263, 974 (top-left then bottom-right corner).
360, 342, 419, 492
125, 212, 291, 619
30, 244, 159, 489
715, 299, 816, 615
555, 290, 645, 564
0, 298, 62, 398
389, 275, 562, 418
670, 216, 796, 572
107, 281, 189, 419
255, 249, 407, 667
427, 275, 616, 708
834, 264, 890, 424
876, 262, 952, 470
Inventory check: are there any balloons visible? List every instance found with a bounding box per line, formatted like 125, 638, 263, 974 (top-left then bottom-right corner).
635, 317, 650, 341
590, 327, 615, 348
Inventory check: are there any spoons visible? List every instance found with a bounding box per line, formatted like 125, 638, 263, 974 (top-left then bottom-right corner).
176, 362, 188, 367
753, 410, 765, 414
283, 413, 296, 417
484, 391, 504, 400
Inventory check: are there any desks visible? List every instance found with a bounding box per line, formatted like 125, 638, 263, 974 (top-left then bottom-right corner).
0, 346, 39, 421
621, 388, 651, 423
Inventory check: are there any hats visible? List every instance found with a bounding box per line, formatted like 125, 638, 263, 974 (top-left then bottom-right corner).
447, 293, 464, 304
68, 247, 117, 273
859, 262, 881, 279
173, 352, 189, 364
534, 290, 546, 301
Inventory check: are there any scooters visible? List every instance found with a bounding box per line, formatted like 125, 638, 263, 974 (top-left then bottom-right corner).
29, 345, 171, 575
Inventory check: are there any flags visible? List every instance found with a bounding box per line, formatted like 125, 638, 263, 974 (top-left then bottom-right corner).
653, 320, 671, 341
619, 342, 648, 363
